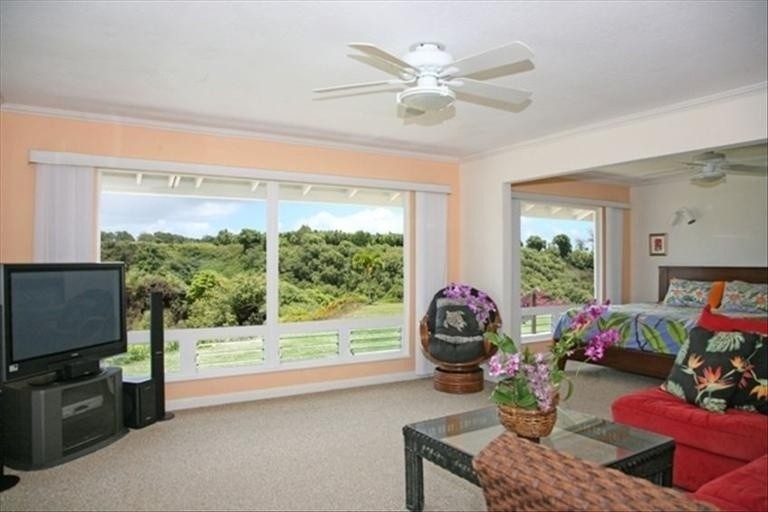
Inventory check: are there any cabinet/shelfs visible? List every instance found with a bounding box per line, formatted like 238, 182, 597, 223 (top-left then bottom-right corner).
1, 367, 130, 470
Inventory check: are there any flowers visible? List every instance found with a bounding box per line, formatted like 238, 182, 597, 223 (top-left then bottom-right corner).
442, 283, 623, 413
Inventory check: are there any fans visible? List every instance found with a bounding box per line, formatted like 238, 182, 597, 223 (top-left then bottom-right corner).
642, 152, 768, 186
312, 41, 535, 110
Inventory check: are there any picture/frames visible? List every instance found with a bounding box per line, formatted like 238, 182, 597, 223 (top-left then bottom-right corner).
649, 234, 666, 256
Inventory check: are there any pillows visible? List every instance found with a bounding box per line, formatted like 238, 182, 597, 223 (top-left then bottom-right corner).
435, 297, 485, 344
660, 277, 768, 314
659, 324, 768, 416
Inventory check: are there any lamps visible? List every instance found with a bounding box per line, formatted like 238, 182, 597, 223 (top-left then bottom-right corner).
669, 207, 696, 226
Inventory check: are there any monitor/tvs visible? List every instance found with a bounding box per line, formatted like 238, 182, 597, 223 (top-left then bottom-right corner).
1, 261, 127, 386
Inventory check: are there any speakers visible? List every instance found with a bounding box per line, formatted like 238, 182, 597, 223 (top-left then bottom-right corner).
151, 291, 174, 420
122, 378, 158, 429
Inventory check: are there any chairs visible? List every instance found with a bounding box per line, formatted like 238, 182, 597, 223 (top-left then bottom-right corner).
420, 286, 502, 393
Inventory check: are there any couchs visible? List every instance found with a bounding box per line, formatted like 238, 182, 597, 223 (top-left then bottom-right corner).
611, 304, 768, 512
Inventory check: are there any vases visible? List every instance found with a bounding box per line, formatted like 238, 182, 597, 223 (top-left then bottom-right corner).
497, 403, 557, 438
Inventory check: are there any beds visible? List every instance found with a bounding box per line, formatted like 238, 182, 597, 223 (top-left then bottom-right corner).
554, 266, 768, 378
